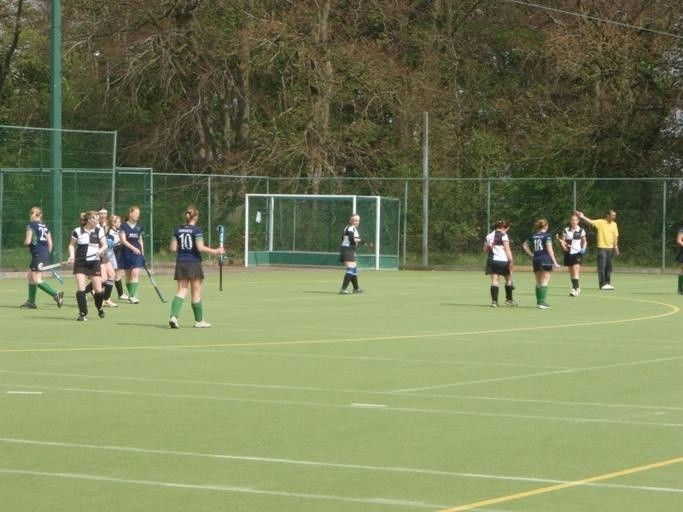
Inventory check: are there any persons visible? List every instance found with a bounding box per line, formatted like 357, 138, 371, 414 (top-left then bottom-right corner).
483, 220, 517, 307
561, 213, 587, 297
522, 219, 560, 308
676, 224, 683, 295
575, 208, 620, 290
338, 214, 374, 294
20, 205, 146, 320
168, 205, 224, 330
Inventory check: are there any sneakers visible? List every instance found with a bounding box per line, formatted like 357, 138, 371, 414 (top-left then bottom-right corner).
601, 284, 614, 289
193, 320, 210, 328
77, 294, 139, 321
339, 287, 364, 295
54, 291, 64, 308
21, 301, 37, 308
491, 299, 519, 308
536, 303, 550, 309
569, 289, 581, 297
169, 317, 179, 328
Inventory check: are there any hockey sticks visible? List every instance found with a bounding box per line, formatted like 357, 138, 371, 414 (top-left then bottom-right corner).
142, 261, 168, 303
216, 225, 224, 291
37, 253, 97, 271
556, 233, 579, 252
